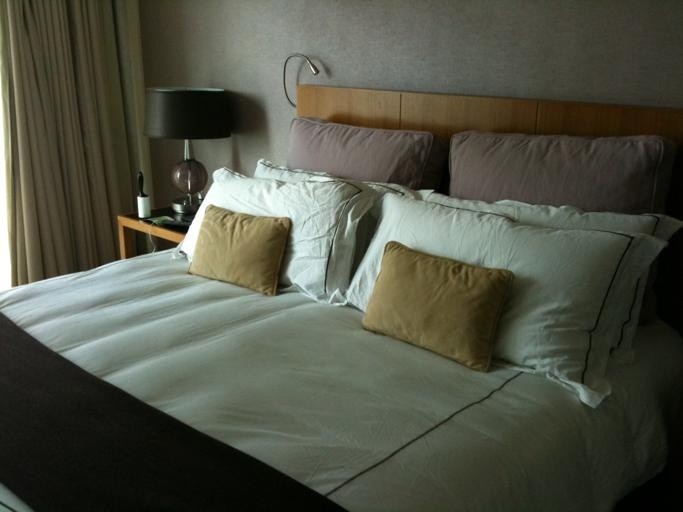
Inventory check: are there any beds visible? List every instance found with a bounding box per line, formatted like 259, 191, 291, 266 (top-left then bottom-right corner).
0, 85, 683, 511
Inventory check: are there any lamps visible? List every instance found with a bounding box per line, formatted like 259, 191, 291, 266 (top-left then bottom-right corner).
141, 87, 234, 215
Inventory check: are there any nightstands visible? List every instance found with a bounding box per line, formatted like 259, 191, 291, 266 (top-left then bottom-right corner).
116, 203, 197, 259
